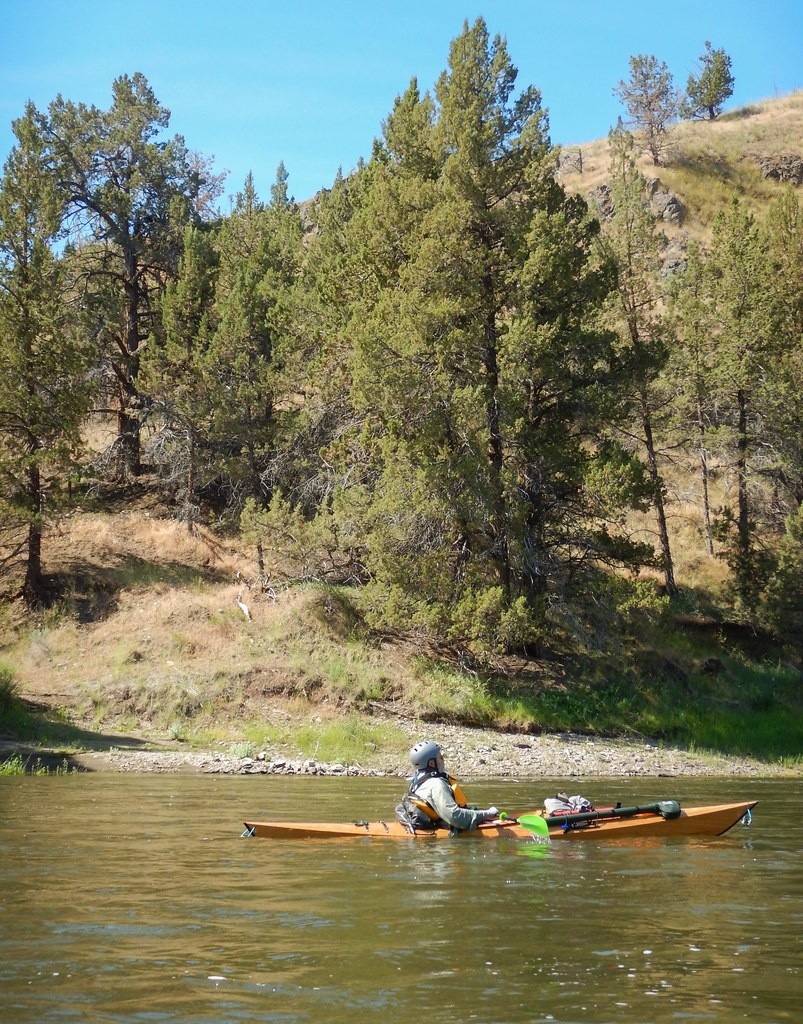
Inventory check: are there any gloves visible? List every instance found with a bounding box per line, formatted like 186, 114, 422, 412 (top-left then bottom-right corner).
483, 806, 499, 821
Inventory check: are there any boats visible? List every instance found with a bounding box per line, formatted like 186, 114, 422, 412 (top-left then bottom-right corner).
240, 801, 758, 840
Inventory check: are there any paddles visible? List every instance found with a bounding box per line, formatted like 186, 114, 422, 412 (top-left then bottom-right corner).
467, 806, 549, 838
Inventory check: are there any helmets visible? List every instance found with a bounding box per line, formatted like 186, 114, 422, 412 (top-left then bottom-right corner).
409, 741, 442, 770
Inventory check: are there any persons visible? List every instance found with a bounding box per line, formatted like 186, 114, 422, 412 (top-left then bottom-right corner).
395, 741, 499, 832
